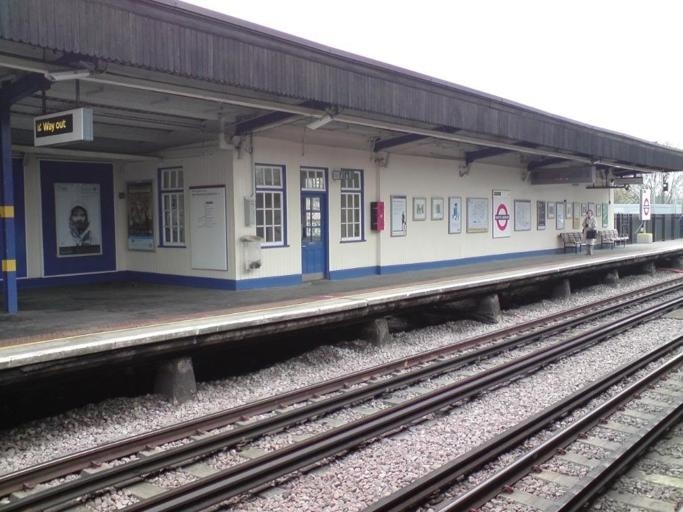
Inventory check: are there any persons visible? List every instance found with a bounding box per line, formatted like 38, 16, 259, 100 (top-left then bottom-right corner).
582, 208, 596, 253
60, 204, 96, 247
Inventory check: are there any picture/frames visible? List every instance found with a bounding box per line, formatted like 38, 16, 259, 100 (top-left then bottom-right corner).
50, 180, 102, 258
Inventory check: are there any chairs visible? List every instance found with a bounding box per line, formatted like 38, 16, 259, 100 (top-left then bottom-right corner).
558, 227, 630, 253
